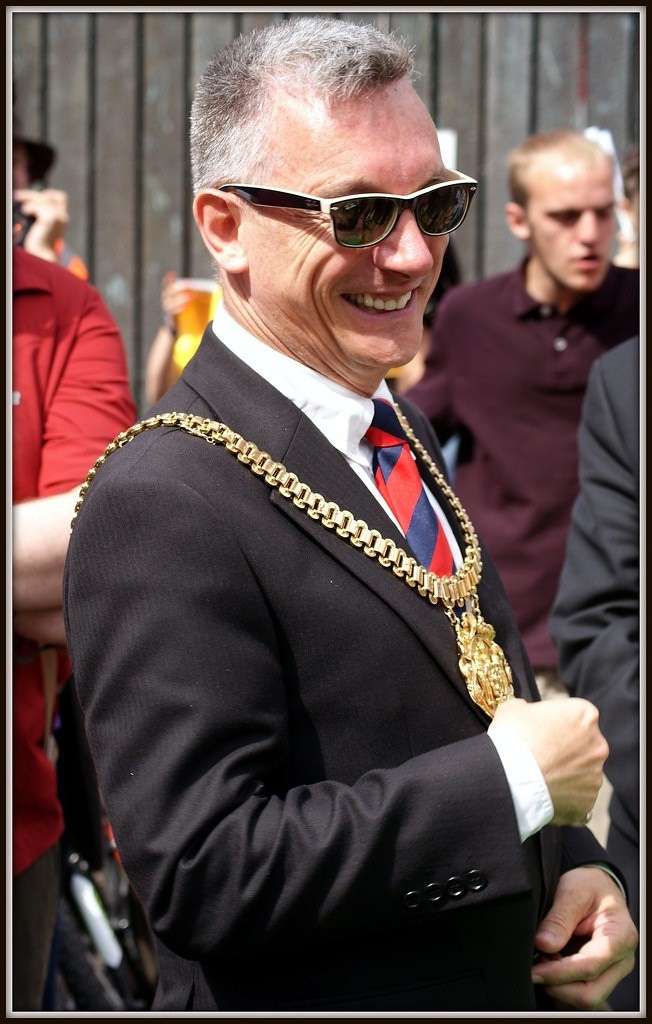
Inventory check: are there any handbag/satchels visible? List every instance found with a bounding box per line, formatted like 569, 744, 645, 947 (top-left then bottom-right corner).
13, 836, 158, 1012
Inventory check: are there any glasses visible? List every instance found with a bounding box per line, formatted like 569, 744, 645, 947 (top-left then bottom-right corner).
221, 166, 481, 253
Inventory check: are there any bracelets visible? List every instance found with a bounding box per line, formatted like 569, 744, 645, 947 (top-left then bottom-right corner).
161, 324, 177, 336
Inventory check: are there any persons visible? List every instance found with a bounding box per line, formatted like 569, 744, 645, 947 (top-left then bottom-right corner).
398, 128, 639, 848
12, 242, 136, 1013
388, 240, 461, 397
12, 115, 88, 282
146, 272, 190, 403
548, 334, 640, 1012
63, 17, 639, 1011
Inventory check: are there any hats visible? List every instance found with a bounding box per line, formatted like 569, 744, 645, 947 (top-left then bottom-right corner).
15, 115, 58, 189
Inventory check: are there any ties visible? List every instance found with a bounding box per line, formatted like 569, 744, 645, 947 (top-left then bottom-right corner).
357, 399, 465, 624
